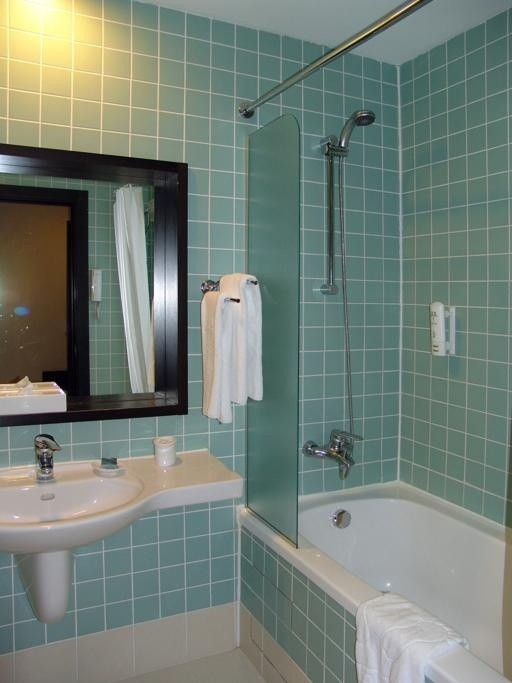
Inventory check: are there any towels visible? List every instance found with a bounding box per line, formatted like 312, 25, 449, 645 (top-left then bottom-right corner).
354, 592, 470, 682
201, 271, 266, 428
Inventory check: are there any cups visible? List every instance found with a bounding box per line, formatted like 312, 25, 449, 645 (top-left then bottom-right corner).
153, 436, 177, 467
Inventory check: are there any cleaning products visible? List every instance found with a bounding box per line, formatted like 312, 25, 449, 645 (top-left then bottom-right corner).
430, 302, 445, 356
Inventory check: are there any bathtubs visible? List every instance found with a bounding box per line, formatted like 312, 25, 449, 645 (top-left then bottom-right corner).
243, 481, 512, 683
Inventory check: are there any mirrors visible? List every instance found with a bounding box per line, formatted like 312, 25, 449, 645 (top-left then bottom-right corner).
0, 141, 190, 427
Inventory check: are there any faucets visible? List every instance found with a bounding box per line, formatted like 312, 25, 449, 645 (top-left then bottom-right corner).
34, 435, 62, 482
328, 430, 363, 470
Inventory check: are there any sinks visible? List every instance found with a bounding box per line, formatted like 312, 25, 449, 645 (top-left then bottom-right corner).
0, 474, 144, 551
